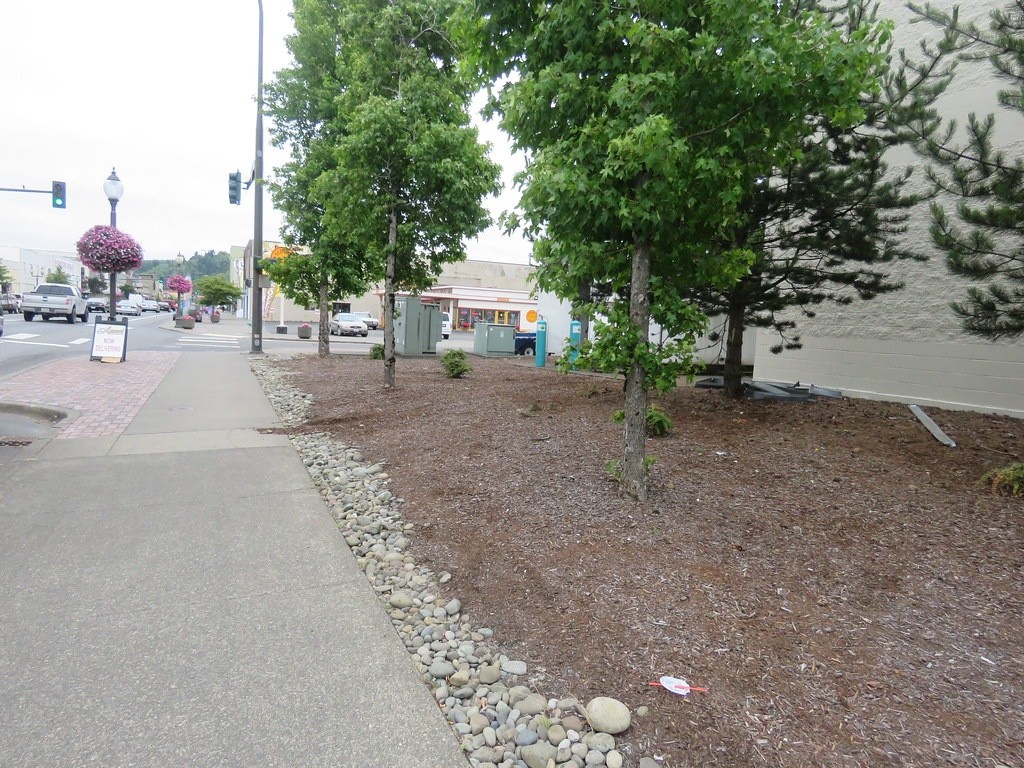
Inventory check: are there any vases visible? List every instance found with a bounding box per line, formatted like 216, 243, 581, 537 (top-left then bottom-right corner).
298, 327, 312, 338
211, 316, 220, 323
196, 316, 202, 322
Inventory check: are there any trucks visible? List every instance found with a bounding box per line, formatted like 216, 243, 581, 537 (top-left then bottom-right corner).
129, 293, 145, 304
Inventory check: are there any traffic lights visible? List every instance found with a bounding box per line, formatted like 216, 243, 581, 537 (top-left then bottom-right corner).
229, 171, 242, 205
159, 276, 164, 283
52, 180, 66, 208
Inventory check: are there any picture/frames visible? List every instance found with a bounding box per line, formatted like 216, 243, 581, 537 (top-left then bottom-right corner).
183, 319, 195, 329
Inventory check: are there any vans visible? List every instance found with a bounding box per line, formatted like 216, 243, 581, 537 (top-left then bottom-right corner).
441, 312, 452, 339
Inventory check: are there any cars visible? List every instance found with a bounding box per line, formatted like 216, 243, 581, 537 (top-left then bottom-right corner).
142, 299, 160, 313
330, 313, 368, 337
190, 304, 199, 314
85, 297, 111, 313
158, 301, 171, 311
115, 300, 142, 316
351, 312, 379, 330
1, 294, 18, 314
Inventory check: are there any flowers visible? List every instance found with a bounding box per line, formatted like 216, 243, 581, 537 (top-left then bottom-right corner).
193, 310, 201, 316
302, 323, 310, 329
183, 314, 194, 320
211, 313, 220, 316
73, 224, 143, 275
166, 274, 192, 294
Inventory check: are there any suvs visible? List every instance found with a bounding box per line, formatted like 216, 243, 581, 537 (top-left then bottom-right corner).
162, 300, 176, 311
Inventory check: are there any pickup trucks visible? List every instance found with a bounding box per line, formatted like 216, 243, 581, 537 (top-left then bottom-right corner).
14, 293, 24, 313
516, 332, 536, 356
21, 283, 89, 324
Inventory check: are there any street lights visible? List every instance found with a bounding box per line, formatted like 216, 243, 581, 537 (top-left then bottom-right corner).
103, 166, 123, 320
76, 277, 79, 287
175, 252, 184, 317
